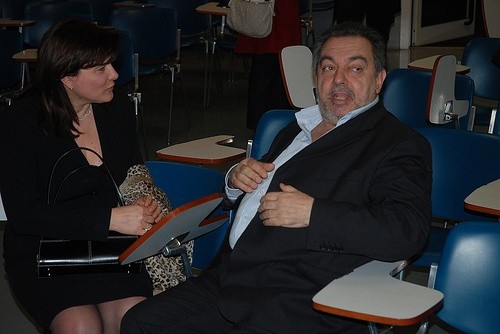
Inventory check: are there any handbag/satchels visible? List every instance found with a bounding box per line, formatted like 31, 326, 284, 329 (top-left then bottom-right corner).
34, 146, 144, 280
226, 0, 276, 38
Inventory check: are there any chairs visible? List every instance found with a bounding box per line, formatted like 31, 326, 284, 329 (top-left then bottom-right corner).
0, 0, 500, 334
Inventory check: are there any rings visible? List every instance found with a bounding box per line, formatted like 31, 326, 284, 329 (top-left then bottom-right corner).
143, 225, 153, 232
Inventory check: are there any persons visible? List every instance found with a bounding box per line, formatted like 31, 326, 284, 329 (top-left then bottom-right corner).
119, 21, 435, 334
331, 0, 402, 73
234, 0, 303, 131
0, 16, 167, 334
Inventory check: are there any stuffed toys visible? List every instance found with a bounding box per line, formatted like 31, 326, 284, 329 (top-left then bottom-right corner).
118, 164, 194, 291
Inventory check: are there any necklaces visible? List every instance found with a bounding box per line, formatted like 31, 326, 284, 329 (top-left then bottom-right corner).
74, 104, 91, 121
